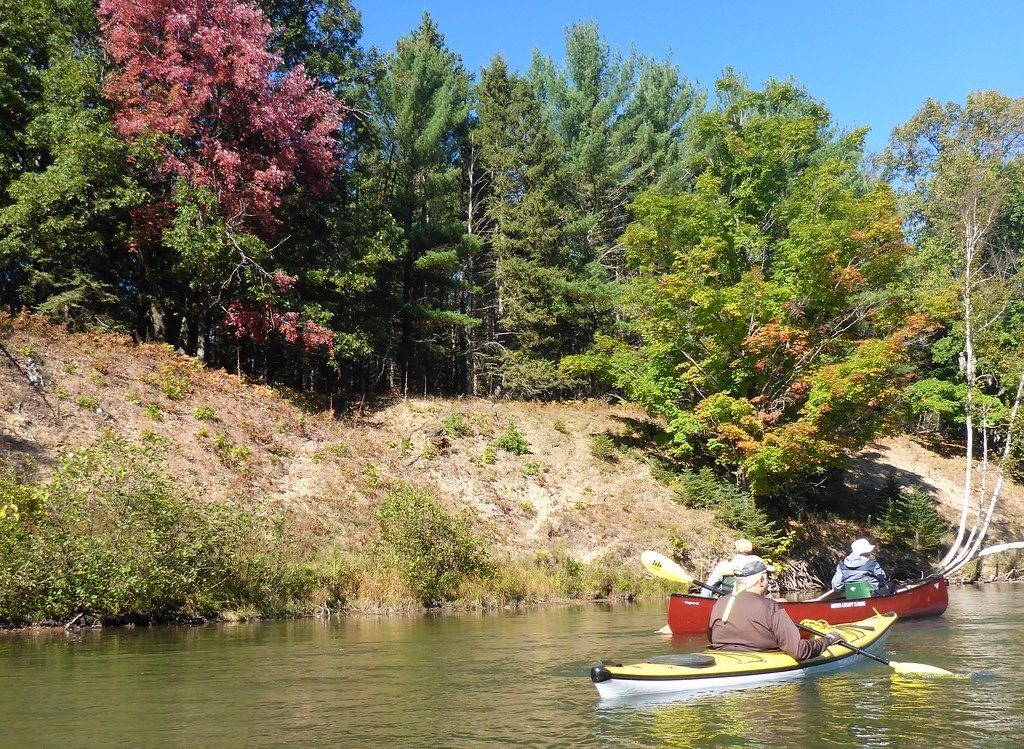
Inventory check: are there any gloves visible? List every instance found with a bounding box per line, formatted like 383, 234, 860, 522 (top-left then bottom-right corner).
825, 631, 845, 646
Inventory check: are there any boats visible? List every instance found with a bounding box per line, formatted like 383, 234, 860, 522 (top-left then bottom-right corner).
668, 571, 949, 635
590, 610, 900, 700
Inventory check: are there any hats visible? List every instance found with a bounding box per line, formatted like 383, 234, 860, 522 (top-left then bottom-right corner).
733, 554, 775, 577
733, 538, 753, 553
848, 539, 875, 557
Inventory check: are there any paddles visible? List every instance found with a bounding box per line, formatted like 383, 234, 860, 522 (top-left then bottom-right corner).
640, 550, 954, 676
800, 589, 835, 602
655, 624, 672, 633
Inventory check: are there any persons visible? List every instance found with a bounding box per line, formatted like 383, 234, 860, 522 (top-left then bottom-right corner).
700, 540, 753, 597
832, 538, 896, 598
704, 553, 844, 661
